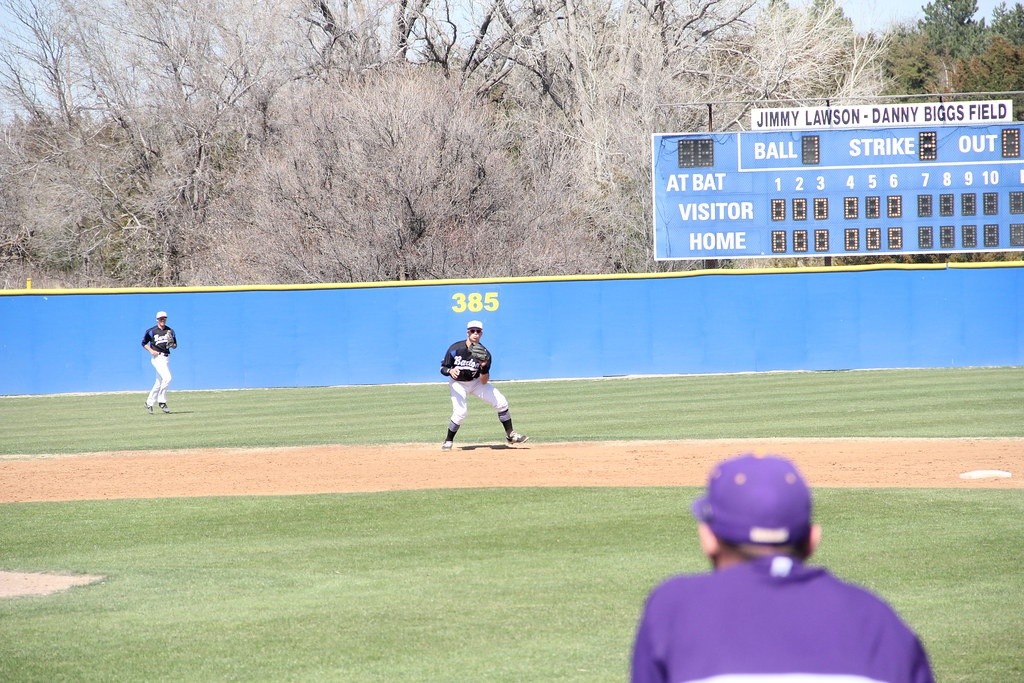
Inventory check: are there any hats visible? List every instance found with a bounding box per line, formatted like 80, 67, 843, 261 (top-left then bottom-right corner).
156, 311, 167, 319
467, 321, 483, 330
694, 455, 809, 549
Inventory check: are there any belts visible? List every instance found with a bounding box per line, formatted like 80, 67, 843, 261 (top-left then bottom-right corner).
159, 353, 168, 356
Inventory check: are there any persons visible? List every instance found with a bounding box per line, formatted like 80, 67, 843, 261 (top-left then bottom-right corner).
141, 311, 177, 416
630, 455, 934, 683
440, 320, 530, 451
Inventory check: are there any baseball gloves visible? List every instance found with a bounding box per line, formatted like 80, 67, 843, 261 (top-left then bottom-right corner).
469, 342, 491, 368
166, 330, 174, 349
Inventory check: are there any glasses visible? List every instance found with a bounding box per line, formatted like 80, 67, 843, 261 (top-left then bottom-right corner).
468, 330, 481, 333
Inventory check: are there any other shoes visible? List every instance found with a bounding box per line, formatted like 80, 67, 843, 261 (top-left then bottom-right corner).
145, 402, 154, 413
159, 403, 169, 413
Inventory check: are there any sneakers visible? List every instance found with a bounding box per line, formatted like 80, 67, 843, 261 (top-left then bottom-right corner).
506, 431, 529, 444
441, 441, 452, 452
